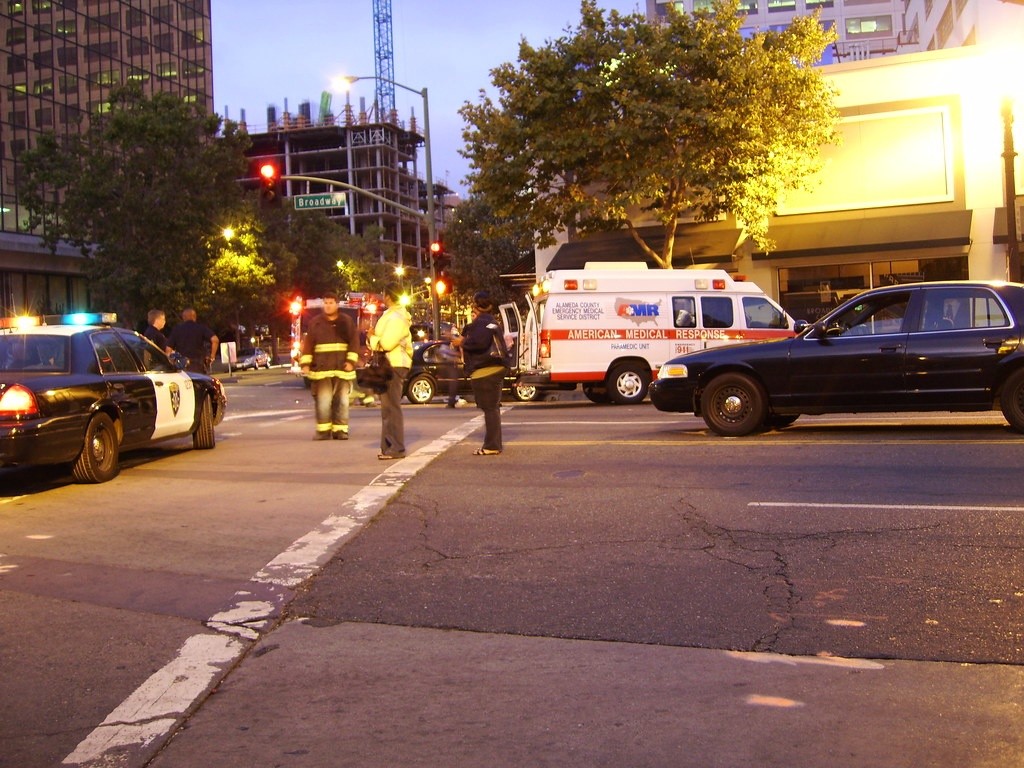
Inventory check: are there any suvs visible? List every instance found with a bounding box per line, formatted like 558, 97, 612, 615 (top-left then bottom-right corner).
648, 280, 1024, 437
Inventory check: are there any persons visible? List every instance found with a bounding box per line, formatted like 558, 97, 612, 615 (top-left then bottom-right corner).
143, 309, 169, 371
451, 292, 511, 456
299, 291, 360, 441
165, 309, 219, 375
364, 282, 413, 460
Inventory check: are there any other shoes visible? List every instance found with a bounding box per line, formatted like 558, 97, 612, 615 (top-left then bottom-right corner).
312, 433, 331, 440
446, 403, 455, 408
377, 452, 405, 459
333, 431, 348, 439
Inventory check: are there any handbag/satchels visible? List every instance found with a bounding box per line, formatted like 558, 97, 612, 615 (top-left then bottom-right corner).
355, 340, 393, 391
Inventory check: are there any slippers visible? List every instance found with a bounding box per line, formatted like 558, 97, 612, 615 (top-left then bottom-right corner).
472, 446, 501, 455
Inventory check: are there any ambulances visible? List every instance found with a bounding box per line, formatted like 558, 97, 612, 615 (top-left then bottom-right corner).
497, 261, 800, 406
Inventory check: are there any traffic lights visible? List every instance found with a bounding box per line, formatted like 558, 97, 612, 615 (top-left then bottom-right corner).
431, 240, 441, 261
258, 156, 280, 202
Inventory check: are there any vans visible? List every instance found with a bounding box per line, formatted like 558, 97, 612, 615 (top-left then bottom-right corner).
289, 291, 385, 390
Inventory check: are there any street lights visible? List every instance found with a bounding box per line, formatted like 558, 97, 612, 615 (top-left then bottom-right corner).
332, 74, 441, 340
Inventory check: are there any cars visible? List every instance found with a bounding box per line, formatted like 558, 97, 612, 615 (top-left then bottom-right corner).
355, 339, 547, 404
0, 311, 227, 484
227, 346, 271, 372
